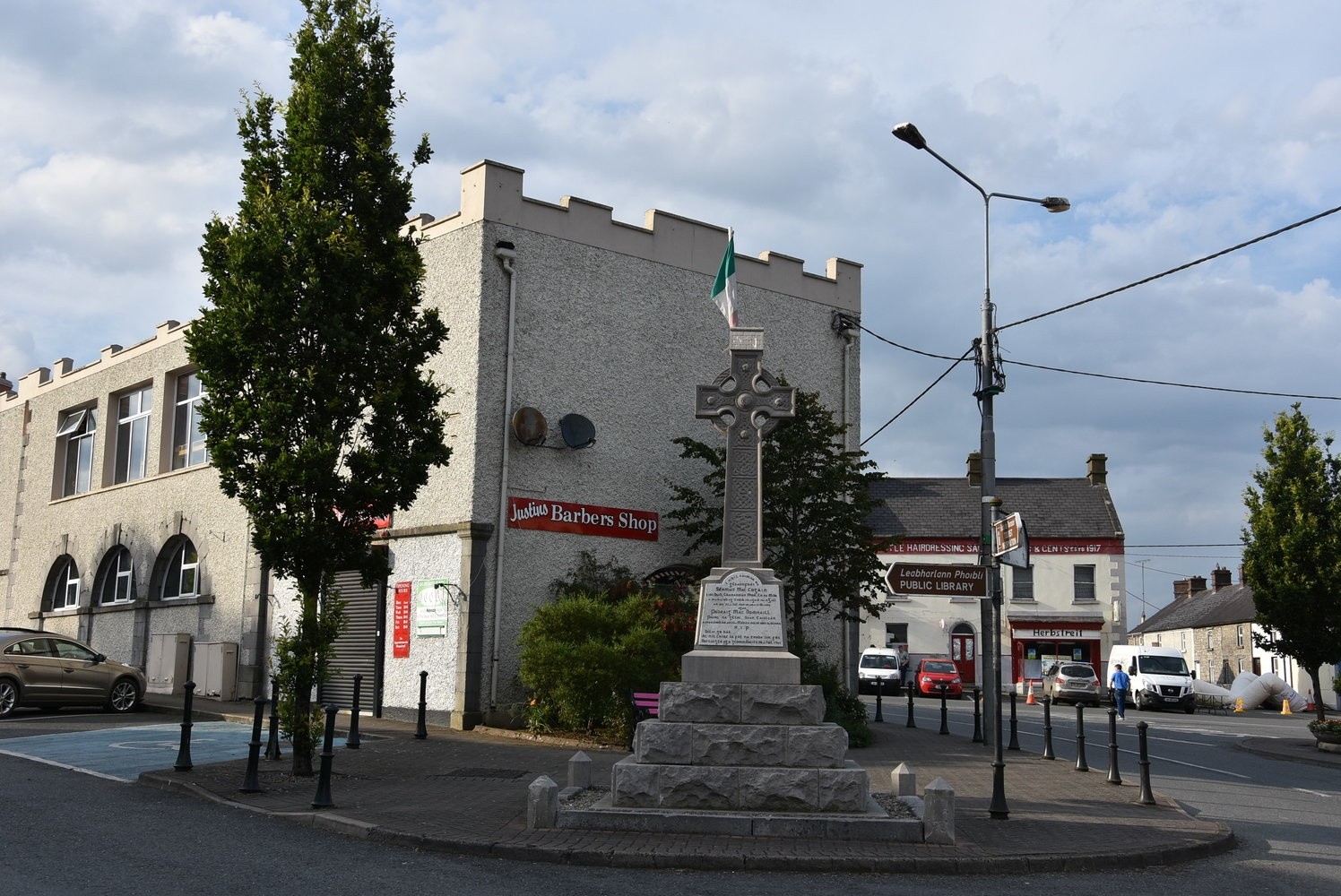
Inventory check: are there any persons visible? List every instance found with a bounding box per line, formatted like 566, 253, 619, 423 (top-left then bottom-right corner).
897, 645, 910, 687
1110, 663, 1130, 721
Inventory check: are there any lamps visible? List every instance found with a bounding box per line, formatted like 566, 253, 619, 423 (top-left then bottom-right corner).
255, 593, 275, 606
434, 583, 466, 603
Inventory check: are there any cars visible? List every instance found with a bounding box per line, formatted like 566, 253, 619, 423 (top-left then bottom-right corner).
913, 658, 962, 700
1041, 660, 1101, 708
0, 626, 147, 714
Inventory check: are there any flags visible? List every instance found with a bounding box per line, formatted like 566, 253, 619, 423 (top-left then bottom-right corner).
709, 233, 738, 333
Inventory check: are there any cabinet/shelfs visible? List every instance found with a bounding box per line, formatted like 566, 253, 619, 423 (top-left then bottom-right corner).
149, 632, 191, 688
191, 641, 238, 699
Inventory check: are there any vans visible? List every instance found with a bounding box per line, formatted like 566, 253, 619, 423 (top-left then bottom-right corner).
1106, 645, 1196, 715
857, 647, 902, 696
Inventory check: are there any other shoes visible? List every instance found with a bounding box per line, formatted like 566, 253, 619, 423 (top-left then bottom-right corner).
1117, 717, 1124, 720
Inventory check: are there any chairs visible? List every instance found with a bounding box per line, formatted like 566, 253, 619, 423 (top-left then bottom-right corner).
925, 664, 932, 669
33, 640, 49, 655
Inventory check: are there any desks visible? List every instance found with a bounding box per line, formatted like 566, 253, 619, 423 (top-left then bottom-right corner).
1195, 692, 1230, 716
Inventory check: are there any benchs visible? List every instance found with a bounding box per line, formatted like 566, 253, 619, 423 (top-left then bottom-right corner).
629, 689, 658, 750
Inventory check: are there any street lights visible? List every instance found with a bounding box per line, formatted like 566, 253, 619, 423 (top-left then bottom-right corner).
892, 122, 1071, 823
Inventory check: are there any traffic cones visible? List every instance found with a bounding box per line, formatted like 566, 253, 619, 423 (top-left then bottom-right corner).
1025, 679, 1040, 705
1280, 699, 1293, 715
1234, 698, 1246, 713
1306, 690, 1314, 711
